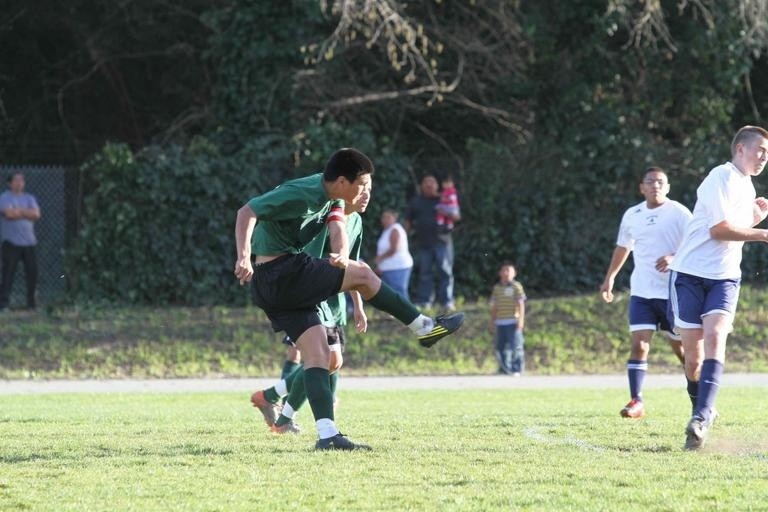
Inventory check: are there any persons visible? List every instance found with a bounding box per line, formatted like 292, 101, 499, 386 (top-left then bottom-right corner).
606, 166, 696, 416
404, 176, 462, 314
277, 319, 346, 415
249, 166, 374, 435
486, 261, 527, 376
372, 200, 414, 300
231, 145, 463, 454
435, 175, 460, 231
0, 169, 40, 317
672, 123, 768, 445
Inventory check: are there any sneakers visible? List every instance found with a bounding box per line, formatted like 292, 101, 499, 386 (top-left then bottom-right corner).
316, 432, 371, 452
269, 421, 305, 435
687, 405, 719, 448
620, 395, 645, 419
418, 313, 463, 349
250, 389, 277, 428
